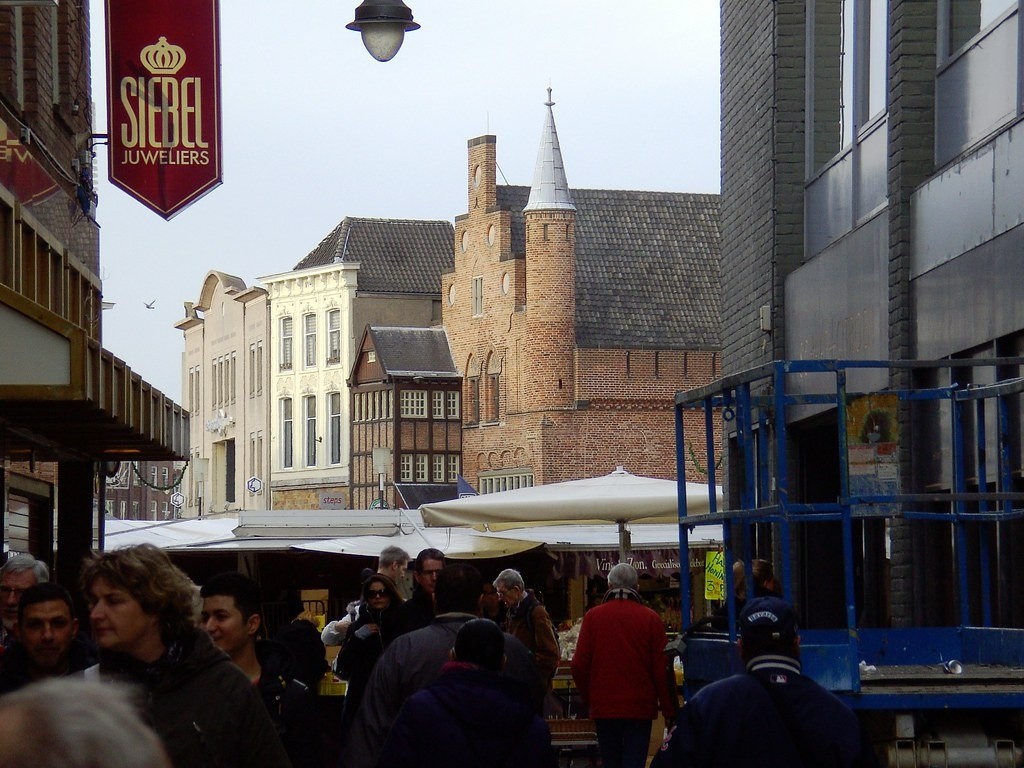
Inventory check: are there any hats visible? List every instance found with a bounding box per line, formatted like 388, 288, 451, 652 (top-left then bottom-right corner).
739, 596, 796, 640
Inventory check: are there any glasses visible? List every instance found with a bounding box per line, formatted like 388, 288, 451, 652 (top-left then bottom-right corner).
0, 585, 26, 602
366, 588, 391, 599
496, 587, 513, 597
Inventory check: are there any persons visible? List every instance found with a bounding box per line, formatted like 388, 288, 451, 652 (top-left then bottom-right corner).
321, 548, 561, 768
570, 562, 680, 768
0, 544, 303, 768
649, 557, 884, 768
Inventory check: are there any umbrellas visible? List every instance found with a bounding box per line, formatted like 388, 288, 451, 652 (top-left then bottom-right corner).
416, 465, 723, 562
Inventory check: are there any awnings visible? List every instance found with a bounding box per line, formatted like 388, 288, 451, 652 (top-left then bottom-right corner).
104, 509, 723, 560
